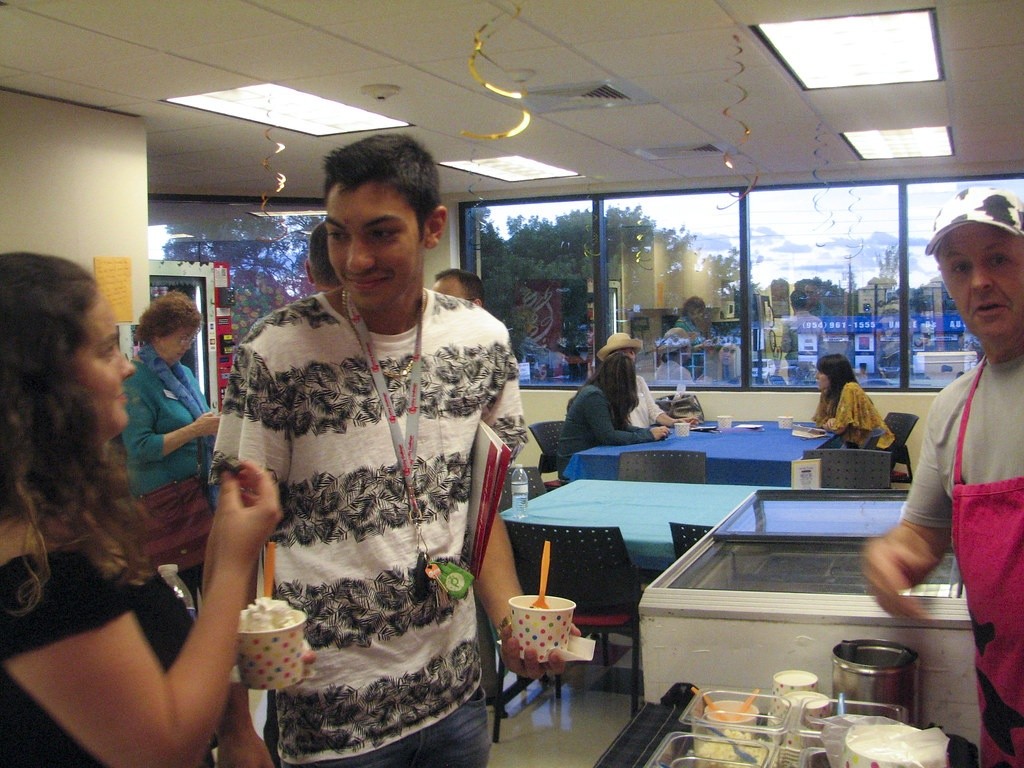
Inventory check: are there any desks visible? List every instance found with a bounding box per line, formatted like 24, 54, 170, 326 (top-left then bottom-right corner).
562, 420, 842, 487
499, 478, 792, 572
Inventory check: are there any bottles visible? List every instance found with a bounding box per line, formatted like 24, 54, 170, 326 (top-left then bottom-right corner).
158, 565, 196, 622
512, 464, 528, 518
152, 286, 168, 298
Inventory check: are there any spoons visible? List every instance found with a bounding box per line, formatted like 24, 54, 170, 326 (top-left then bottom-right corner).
686, 715, 759, 762
532, 541, 551, 609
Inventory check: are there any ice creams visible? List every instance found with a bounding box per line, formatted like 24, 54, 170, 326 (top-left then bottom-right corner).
237, 597, 296, 632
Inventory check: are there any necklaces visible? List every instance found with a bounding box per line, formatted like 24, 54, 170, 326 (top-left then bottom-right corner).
341, 289, 427, 377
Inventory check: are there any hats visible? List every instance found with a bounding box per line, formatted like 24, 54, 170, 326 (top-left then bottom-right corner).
925, 185, 1024, 256
596, 333, 643, 362
658, 328, 698, 354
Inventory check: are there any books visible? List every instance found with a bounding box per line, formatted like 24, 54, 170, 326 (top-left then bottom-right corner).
468, 421, 511, 581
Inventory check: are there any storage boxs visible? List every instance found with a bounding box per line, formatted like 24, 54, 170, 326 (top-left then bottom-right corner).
643, 687, 909, 768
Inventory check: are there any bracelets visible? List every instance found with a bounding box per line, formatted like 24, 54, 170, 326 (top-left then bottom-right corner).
497, 616, 511, 636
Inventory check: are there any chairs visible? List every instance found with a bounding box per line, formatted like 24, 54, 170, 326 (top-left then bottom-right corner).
802, 412, 920, 490
492, 449, 714, 744
528, 419, 564, 491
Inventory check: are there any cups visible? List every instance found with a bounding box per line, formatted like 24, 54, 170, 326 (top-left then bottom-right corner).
235, 608, 306, 690
718, 416, 732, 430
509, 595, 576, 664
842, 724, 922, 768
674, 423, 690, 437
777, 691, 831, 768
777, 416, 794, 429
704, 701, 759, 725
766, 670, 819, 738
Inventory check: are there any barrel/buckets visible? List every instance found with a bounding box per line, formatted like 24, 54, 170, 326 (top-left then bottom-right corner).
832, 638, 919, 727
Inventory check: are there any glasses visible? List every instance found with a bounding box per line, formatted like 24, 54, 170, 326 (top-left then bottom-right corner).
173, 333, 196, 345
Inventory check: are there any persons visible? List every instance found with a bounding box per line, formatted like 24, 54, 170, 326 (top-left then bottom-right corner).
813, 353, 898, 472
506, 305, 569, 370
119, 290, 222, 611
202, 134, 583, 768
0, 251, 286, 768
864, 186, 1024, 768
656, 296, 740, 382
597, 332, 699, 428
558, 352, 670, 483
306, 222, 342, 292
435, 269, 486, 306
773, 283, 827, 360
